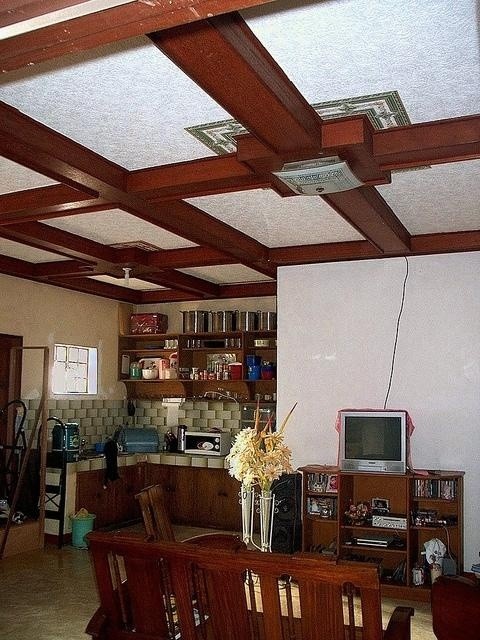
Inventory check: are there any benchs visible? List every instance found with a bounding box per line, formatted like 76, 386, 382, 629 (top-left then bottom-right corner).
79, 533, 415, 639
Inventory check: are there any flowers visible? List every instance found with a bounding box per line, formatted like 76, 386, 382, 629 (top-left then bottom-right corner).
250, 436, 302, 491
226, 424, 264, 491
346, 503, 369, 519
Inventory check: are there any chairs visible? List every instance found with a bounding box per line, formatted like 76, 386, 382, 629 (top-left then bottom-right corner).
431, 574, 480, 639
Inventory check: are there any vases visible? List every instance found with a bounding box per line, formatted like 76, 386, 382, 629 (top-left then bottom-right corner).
355, 521, 364, 525
239, 486, 255, 546
260, 497, 276, 553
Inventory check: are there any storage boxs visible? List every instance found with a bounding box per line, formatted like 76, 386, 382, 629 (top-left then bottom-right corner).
127, 313, 168, 334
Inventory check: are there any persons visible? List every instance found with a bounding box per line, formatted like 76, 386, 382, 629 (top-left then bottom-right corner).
330, 476, 337, 490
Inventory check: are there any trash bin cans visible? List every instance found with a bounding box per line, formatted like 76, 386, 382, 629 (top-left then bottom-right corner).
68, 514, 96, 546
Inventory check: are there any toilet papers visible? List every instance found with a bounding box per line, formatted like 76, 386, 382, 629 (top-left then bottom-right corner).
161, 402, 180, 426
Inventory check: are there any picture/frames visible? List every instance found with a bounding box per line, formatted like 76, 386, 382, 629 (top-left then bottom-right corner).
326, 474, 338, 493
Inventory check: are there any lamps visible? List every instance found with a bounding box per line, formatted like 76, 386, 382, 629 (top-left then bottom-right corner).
272, 156, 366, 197
123, 266, 132, 286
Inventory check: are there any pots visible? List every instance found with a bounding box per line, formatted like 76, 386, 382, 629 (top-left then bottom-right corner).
209, 309, 233, 332
180, 309, 208, 331
231, 310, 257, 331
256, 309, 276, 331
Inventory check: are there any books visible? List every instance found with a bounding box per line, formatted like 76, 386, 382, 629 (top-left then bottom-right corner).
413, 478, 457, 499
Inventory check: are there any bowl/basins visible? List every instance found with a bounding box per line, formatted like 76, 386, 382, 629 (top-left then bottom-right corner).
162, 368, 177, 380
253, 340, 270, 347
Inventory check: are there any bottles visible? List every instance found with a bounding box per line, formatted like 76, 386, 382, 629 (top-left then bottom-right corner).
130, 362, 140, 379
185, 338, 200, 348
190, 360, 229, 380
225, 338, 241, 348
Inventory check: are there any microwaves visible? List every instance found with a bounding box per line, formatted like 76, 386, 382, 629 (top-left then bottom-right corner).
185, 430, 230, 456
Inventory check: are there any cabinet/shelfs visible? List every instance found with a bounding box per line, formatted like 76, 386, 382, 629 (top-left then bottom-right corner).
77, 462, 258, 531
120, 333, 277, 400
301, 462, 466, 605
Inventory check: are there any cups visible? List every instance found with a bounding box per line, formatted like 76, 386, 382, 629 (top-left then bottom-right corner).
272, 393, 277, 401
265, 395, 271, 400
254, 394, 263, 401
412, 568, 424, 585
165, 340, 177, 349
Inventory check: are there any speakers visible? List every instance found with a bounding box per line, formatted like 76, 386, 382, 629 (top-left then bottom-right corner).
268, 472, 302, 554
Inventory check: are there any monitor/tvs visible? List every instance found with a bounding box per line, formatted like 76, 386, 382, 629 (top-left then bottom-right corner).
339, 411, 407, 474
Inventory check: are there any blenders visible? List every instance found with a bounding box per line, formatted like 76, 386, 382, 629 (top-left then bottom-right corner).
163, 429, 177, 452
140, 358, 161, 380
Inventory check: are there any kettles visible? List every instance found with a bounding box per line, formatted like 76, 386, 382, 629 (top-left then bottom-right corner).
177, 425, 187, 454
158, 359, 169, 378
